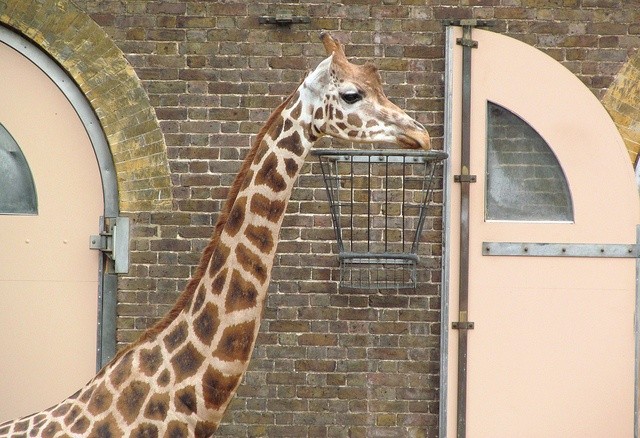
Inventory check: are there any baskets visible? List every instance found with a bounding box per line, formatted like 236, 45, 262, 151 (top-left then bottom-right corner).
310, 149, 449, 289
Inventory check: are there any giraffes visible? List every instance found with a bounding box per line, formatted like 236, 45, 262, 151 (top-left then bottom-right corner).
0, 29, 431, 438
